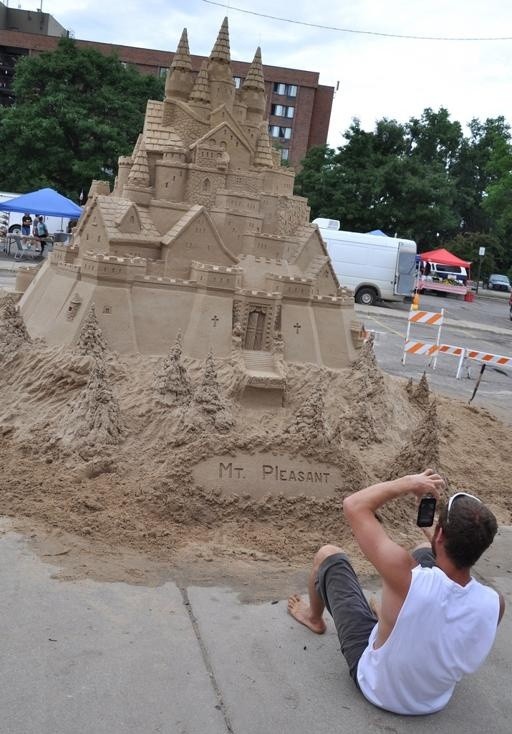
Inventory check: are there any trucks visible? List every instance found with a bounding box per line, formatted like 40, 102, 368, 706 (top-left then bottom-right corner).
318, 227, 418, 306
0, 192, 73, 239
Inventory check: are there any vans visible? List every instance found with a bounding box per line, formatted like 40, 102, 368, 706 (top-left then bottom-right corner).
422, 261, 468, 283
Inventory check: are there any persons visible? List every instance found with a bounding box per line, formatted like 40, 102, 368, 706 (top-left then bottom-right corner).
288, 466, 505, 716
37, 216, 49, 253
22, 212, 33, 249
424, 260, 431, 275
30, 213, 41, 251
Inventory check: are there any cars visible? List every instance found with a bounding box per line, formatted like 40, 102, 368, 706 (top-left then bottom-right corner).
483, 273, 512, 293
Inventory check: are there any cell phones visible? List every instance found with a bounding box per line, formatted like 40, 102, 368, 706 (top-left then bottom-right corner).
417, 497, 436, 528
